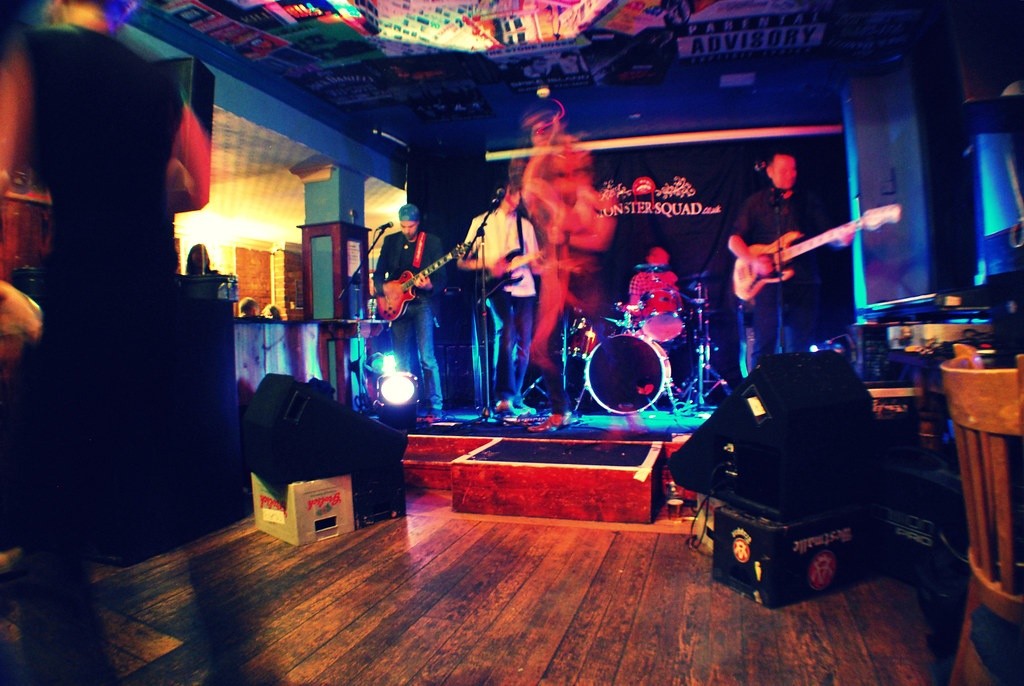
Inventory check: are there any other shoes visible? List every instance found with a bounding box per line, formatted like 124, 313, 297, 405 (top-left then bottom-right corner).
418, 411, 443, 423
529, 414, 569, 432
496, 400, 538, 417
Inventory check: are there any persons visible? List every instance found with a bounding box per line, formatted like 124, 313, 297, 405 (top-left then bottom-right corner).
458, 178, 537, 414
370, 204, 447, 420
0, 0, 289, 686
731, 153, 852, 374
522, 101, 682, 433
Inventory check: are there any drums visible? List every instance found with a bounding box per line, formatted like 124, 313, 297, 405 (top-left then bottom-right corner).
584, 333, 671, 414
554, 312, 597, 360
638, 287, 687, 342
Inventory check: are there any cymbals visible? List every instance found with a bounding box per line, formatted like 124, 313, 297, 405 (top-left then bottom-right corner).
634, 264, 674, 273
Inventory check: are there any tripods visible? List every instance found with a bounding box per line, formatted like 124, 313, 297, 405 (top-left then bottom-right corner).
677, 282, 733, 410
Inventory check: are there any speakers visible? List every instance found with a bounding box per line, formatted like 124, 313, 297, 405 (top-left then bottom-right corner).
240, 371, 409, 481
668, 350, 874, 525
157, 55, 215, 142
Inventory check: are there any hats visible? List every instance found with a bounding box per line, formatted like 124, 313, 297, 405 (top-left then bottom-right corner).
398, 204, 419, 222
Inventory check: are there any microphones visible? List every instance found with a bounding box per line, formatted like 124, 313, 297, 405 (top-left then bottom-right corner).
489, 188, 505, 211
376, 222, 393, 231
774, 187, 782, 212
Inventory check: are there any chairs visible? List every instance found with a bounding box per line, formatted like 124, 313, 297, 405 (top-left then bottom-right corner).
936, 341, 1024, 685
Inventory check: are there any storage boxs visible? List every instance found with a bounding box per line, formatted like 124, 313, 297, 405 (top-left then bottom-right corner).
247, 473, 355, 547
710, 505, 861, 611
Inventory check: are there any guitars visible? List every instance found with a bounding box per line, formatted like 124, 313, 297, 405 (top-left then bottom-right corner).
733, 202, 903, 301
376, 241, 476, 323
479, 248, 543, 299
